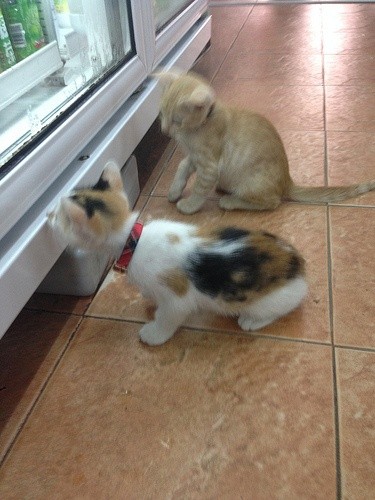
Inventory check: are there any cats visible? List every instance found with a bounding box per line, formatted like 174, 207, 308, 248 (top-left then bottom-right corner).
147, 69, 375, 216
45, 160, 308, 346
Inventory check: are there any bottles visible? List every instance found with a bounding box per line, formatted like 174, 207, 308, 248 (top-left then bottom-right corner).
0, 6, 18, 76
55, 1, 70, 32
1, 0, 45, 65
